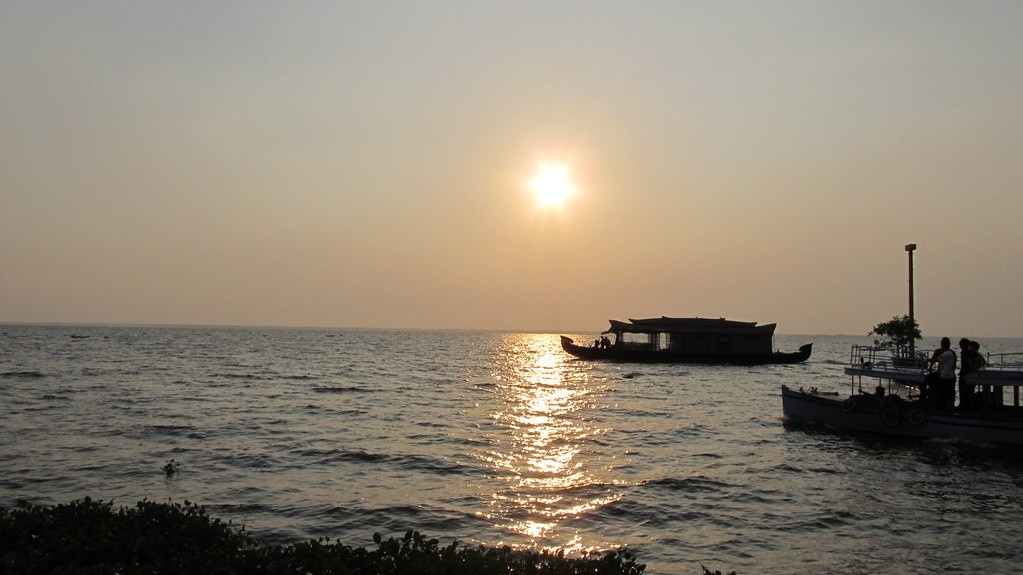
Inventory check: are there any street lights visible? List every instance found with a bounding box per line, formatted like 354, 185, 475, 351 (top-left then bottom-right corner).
904, 244, 919, 361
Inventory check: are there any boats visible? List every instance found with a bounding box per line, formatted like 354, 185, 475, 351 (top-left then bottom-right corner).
560, 316, 813, 365
781, 244, 1023, 447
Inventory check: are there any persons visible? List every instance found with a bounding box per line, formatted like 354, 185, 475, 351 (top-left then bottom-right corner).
928, 336, 957, 409
959, 338, 987, 406
592, 336, 610, 349
